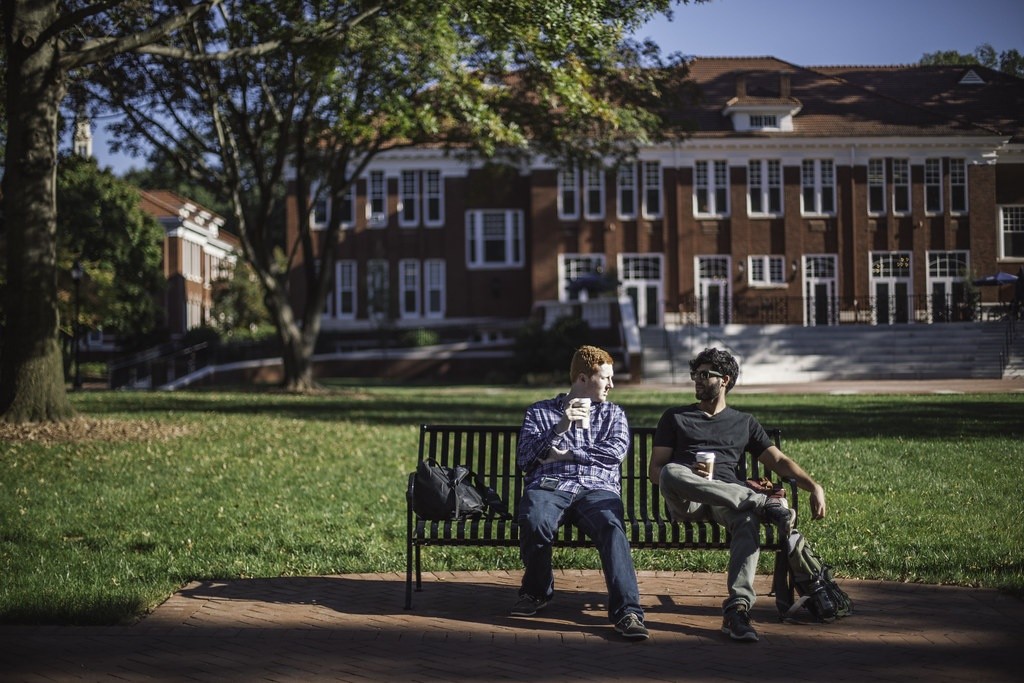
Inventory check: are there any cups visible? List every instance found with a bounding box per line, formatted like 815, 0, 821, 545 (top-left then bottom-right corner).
571, 398, 592, 429
695, 452, 715, 481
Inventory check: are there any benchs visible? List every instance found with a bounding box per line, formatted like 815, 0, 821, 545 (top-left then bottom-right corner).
404, 422, 798, 613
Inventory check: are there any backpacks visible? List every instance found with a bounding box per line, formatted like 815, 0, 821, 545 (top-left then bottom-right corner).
412, 457, 513, 521
769, 528, 855, 625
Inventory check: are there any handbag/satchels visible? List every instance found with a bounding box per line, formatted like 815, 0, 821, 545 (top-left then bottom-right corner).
746, 477, 786, 497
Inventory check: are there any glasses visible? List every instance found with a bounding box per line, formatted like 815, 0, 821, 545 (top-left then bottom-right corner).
690, 369, 724, 380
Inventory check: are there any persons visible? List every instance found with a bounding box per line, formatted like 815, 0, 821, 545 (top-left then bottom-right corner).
649, 348, 826, 641
510, 346, 650, 639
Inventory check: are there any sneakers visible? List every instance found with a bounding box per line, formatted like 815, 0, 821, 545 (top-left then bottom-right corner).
721, 604, 759, 641
614, 612, 650, 638
760, 498, 796, 533
511, 593, 547, 615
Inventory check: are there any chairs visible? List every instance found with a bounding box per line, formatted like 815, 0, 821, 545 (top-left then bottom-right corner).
987, 298, 1023, 321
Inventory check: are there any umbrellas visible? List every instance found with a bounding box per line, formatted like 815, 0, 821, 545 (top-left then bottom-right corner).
972, 272, 1019, 317
